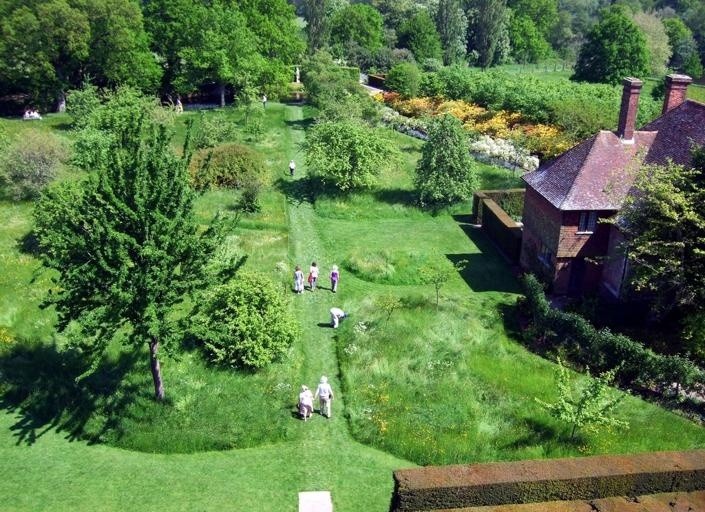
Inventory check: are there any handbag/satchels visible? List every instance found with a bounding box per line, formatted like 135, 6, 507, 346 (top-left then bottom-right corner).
307, 273, 312, 283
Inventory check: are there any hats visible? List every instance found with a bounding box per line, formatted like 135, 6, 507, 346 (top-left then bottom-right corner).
319, 376, 328, 383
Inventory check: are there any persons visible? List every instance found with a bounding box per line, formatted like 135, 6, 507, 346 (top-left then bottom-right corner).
298, 383, 314, 422
330, 307, 349, 329
293, 265, 304, 295
309, 261, 319, 292
261, 95, 267, 108
329, 265, 339, 293
289, 160, 296, 176
314, 376, 334, 420
